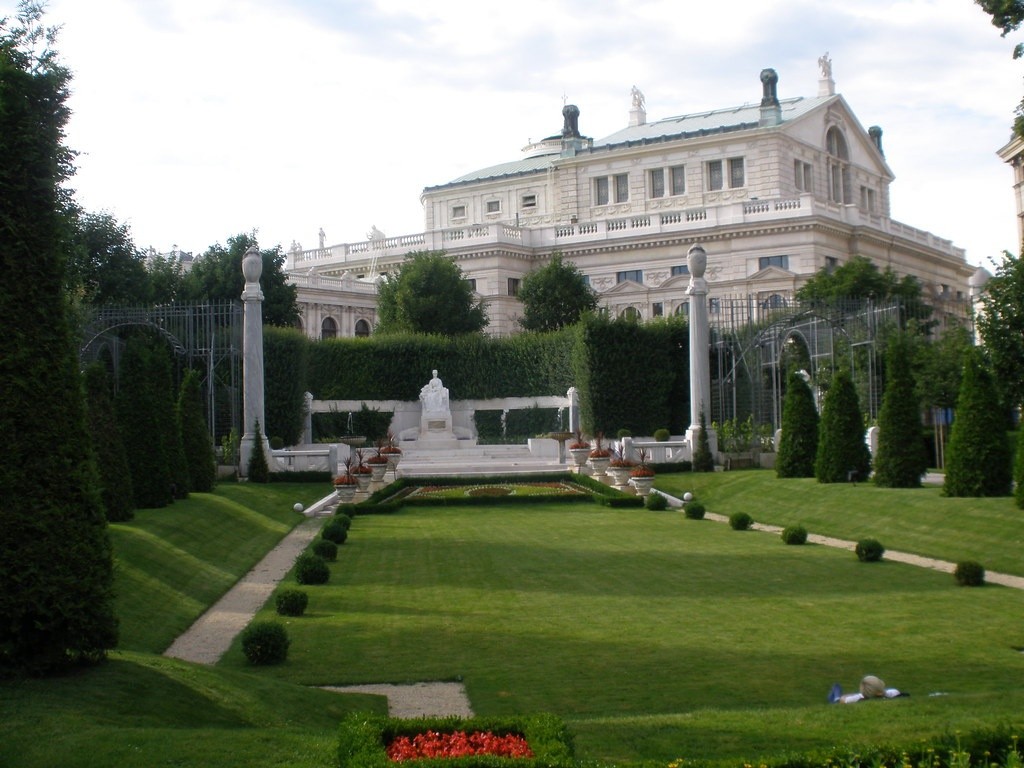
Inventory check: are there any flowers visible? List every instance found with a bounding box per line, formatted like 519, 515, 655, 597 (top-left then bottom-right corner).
332, 475, 360, 485
590, 449, 611, 458
609, 460, 634, 467
630, 464, 655, 476
568, 442, 591, 449
380, 446, 401, 453
350, 466, 373, 474
367, 456, 389, 464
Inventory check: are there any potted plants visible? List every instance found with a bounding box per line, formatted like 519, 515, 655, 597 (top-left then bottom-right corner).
332, 456, 361, 504
350, 446, 374, 493
569, 425, 593, 467
364, 437, 389, 482
381, 428, 402, 471
589, 431, 611, 476
607, 443, 634, 486
631, 447, 656, 497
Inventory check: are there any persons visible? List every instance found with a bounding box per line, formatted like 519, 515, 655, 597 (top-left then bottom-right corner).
427, 370, 443, 411
840, 676, 907, 706
